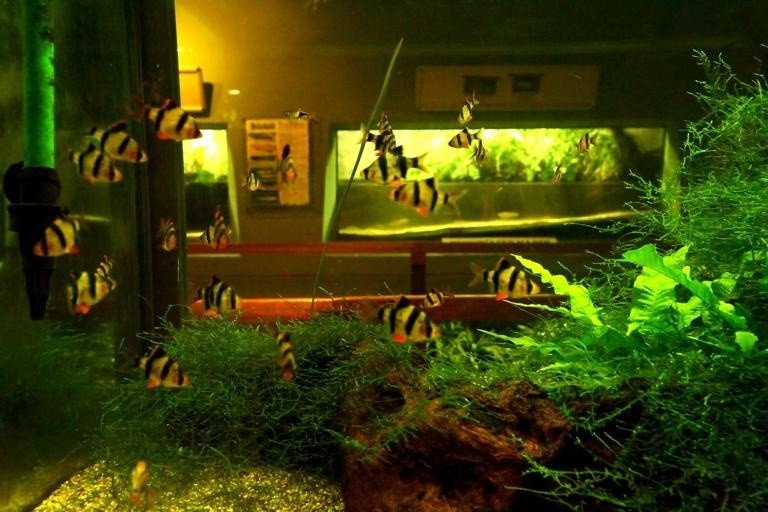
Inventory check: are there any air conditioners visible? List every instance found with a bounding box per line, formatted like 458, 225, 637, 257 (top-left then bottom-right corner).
413, 64, 600, 112
